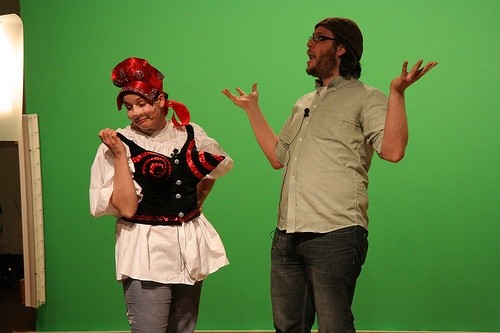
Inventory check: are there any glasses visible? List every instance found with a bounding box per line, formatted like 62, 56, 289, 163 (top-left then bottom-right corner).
309, 33, 339, 43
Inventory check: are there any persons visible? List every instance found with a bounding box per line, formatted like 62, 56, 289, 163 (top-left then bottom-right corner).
221, 17, 438, 333
89, 57, 234, 333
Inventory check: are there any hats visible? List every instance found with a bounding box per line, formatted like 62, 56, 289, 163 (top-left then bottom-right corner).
315, 18, 363, 61
111, 57, 190, 127
